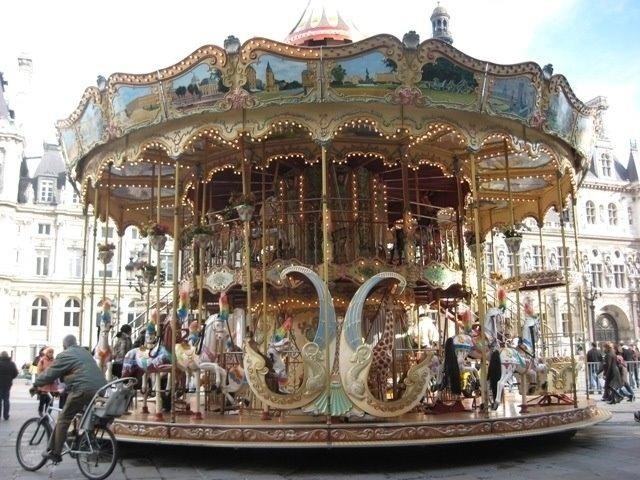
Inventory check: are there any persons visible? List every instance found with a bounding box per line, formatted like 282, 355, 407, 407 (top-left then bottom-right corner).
22, 347, 70, 416
0, 351, 18, 420
29, 333, 108, 463
577, 339, 640, 404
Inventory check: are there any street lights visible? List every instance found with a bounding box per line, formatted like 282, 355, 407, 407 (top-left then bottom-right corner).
583, 282, 602, 343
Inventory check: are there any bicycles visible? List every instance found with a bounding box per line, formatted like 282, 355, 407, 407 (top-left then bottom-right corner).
16, 377, 138, 480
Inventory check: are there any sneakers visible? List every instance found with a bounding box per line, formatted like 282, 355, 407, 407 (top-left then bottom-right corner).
599, 394, 640, 422
2, 412, 43, 420
40, 451, 61, 462
